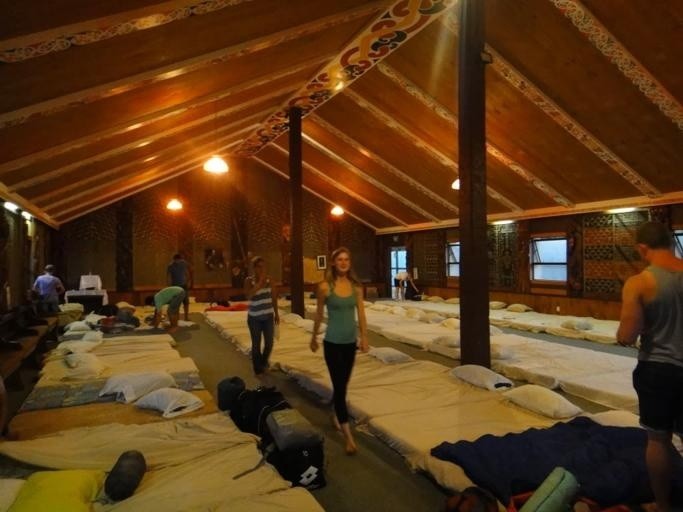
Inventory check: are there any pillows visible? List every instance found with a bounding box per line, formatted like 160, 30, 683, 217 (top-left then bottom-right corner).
0, 289, 642, 512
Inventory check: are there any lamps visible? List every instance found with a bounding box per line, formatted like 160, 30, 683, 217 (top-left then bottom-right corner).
452, 179, 460, 190
203, 99, 229, 173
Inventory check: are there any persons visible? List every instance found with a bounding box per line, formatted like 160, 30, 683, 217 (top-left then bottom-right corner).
394, 273, 418, 301
245, 255, 280, 378
167, 254, 194, 320
309, 247, 368, 454
144, 286, 186, 334
617, 219, 683, 512
33, 264, 65, 340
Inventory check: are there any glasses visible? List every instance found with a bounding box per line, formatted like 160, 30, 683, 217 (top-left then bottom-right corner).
167, 198, 183, 211
203, 99, 229, 173
452, 179, 460, 190
330, 205, 345, 215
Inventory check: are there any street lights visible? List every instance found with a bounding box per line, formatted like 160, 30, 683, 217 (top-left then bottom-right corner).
230, 387, 325, 491
